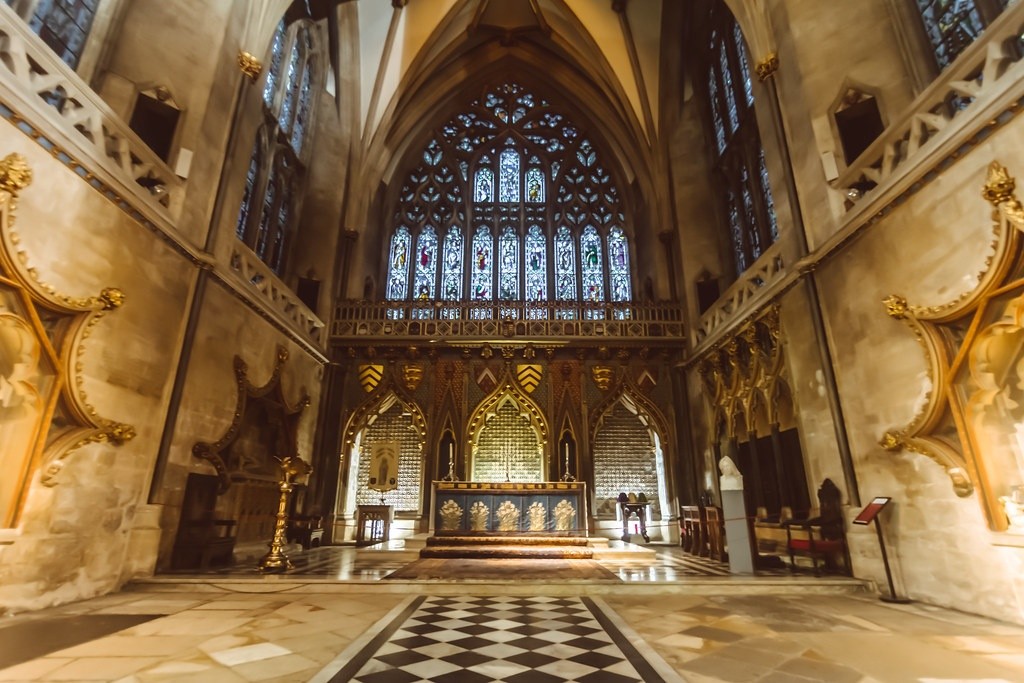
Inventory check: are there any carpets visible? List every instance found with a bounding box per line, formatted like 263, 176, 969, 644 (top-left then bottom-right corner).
378, 558, 624, 581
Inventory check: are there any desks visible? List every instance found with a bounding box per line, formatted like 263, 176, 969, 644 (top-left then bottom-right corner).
356, 505, 394, 547
621, 501, 650, 544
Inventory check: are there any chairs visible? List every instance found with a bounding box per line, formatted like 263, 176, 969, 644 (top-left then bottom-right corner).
780, 478, 854, 578
171, 473, 238, 570
287, 488, 323, 549
